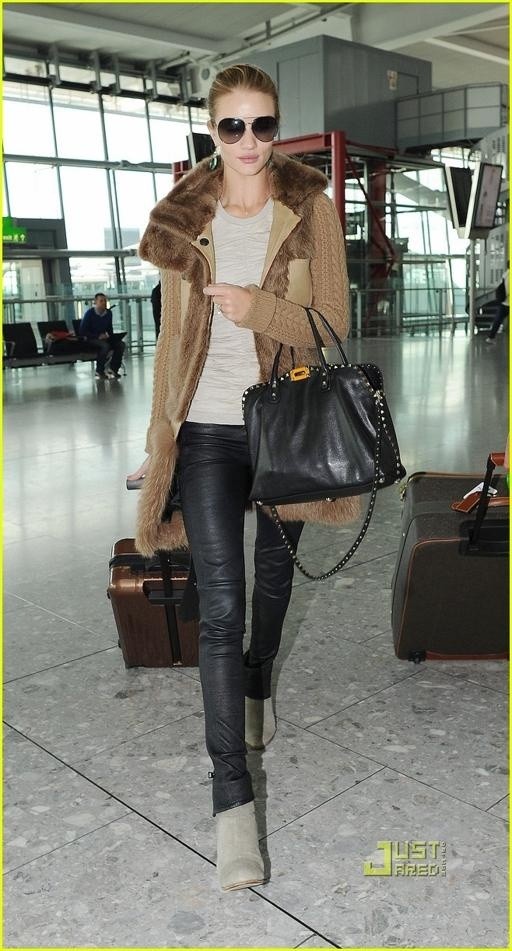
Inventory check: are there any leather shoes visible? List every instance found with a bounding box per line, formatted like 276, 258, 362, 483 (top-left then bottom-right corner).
215, 800, 268, 893
244, 696, 277, 751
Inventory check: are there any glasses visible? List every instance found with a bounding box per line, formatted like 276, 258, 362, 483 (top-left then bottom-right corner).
217, 115, 277, 144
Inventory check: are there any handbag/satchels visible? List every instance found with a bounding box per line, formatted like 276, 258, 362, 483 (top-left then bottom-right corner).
241, 363, 407, 507
46, 332, 75, 357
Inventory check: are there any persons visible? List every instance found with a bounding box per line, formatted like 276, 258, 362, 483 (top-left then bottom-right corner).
135, 63, 362, 893
78, 294, 126, 377
485, 260, 510, 344
151, 280, 161, 338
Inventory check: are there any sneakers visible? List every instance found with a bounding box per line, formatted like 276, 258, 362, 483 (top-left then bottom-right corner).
107, 367, 122, 378
95, 371, 108, 379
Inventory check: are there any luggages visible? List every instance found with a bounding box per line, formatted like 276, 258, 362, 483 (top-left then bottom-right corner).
391, 453, 510, 664
107, 475, 201, 669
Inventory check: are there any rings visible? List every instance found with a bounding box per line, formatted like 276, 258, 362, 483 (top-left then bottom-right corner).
217, 304, 222, 315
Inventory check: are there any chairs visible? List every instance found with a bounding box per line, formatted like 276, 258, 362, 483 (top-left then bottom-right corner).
3, 319, 128, 377
